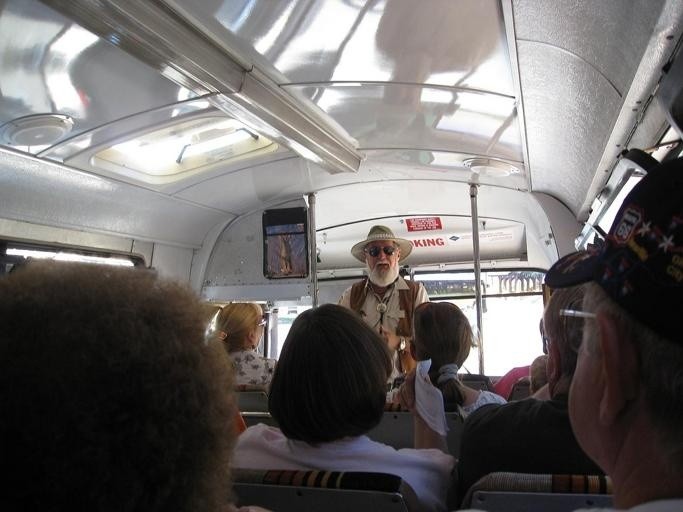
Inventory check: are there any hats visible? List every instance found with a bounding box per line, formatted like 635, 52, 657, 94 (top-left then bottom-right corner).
544, 156, 681, 345
350, 226, 412, 263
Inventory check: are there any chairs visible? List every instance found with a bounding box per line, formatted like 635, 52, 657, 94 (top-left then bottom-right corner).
456, 468, 614, 510
228, 469, 423, 510
233, 371, 543, 465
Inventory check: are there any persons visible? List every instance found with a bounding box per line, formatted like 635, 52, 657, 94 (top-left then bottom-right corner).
1, 258, 269, 511
461, 282, 604, 477
206, 303, 278, 392
545, 158, 683, 512
337, 225, 429, 404
228, 303, 456, 512
388, 302, 508, 420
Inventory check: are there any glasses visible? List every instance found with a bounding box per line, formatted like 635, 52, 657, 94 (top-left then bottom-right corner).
559, 294, 597, 352
257, 318, 267, 327
363, 246, 396, 257
